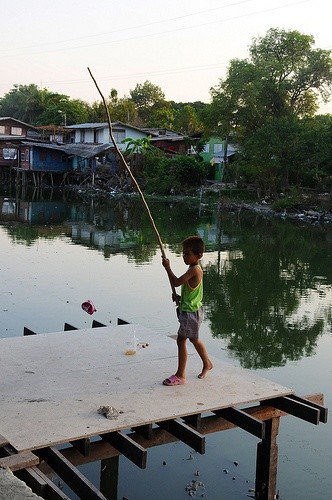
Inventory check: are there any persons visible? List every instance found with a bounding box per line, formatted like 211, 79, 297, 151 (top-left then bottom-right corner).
161, 235, 213, 387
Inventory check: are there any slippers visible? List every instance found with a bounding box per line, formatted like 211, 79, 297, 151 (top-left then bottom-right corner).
81, 299, 96, 315
163, 375, 185, 385
